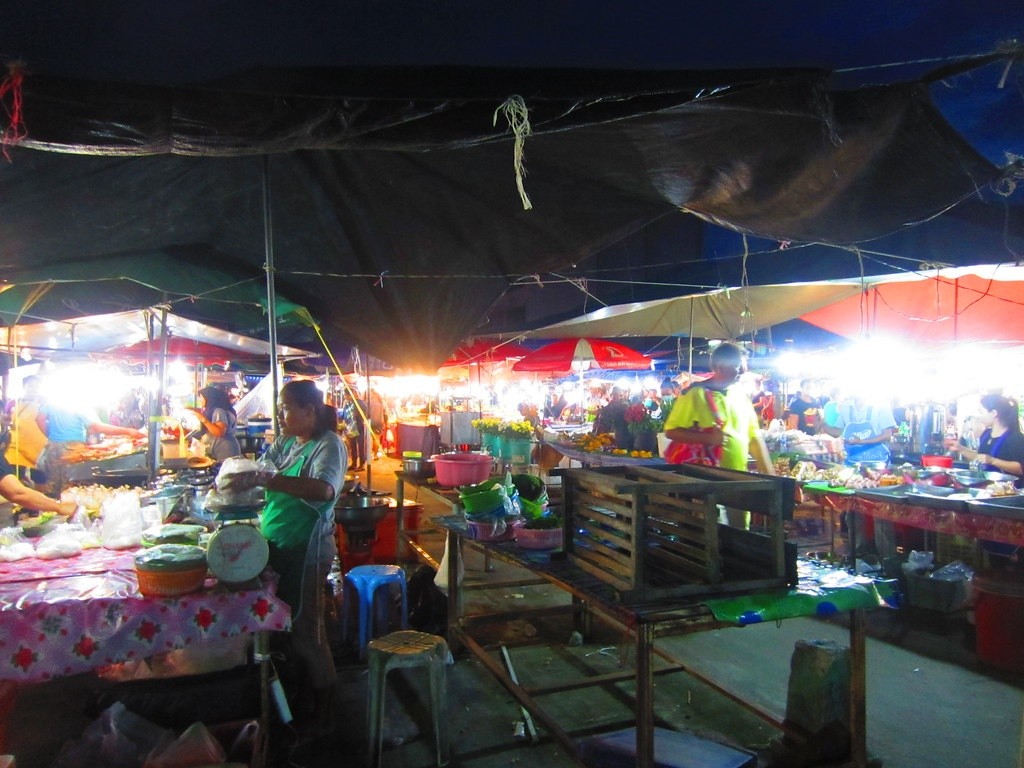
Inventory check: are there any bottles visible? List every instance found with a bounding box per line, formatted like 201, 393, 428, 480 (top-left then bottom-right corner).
944, 427, 958, 460
779, 434, 788, 454
898, 421, 909, 444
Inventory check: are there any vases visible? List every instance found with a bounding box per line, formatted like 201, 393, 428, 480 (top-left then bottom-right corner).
611, 423, 629, 450
479, 432, 532, 465
632, 426, 655, 452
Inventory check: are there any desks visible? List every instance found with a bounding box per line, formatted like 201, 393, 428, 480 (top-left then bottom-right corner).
394, 468, 535, 621
431, 512, 883, 768
804, 465, 1024, 574
0, 489, 292, 768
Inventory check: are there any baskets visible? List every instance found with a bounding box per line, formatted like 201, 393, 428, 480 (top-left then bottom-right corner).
141, 537, 202, 550
131, 565, 207, 595
459, 473, 563, 549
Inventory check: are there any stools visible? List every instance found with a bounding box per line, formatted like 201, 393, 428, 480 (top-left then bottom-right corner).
342, 565, 408, 658
366, 630, 454, 768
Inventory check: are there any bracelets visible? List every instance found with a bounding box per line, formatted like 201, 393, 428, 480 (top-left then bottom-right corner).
201, 417, 206, 424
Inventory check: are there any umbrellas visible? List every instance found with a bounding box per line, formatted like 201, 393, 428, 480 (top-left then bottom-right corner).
521, 265, 891, 386
0, 305, 320, 445
420, 335, 533, 448
798, 265, 1024, 361
0, 251, 314, 481
513, 337, 653, 420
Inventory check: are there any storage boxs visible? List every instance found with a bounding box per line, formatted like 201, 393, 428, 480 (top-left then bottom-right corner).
901, 560, 976, 612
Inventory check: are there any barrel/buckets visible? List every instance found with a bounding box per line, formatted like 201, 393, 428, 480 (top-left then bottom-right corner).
236, 418, 271, 451
152, 482, 198, 523
973, 589, 1024, 674
909, 401, 948, 456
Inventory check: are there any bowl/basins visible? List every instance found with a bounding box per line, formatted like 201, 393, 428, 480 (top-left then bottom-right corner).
401, 456, 436, 478
947, 468, 1020, 486
806, 550, 842, 567
923, 455, 954, 467
460, 474, 551, 542
335, 496, 390, 524
431, 453, 493, 487
140, 526, 207, 548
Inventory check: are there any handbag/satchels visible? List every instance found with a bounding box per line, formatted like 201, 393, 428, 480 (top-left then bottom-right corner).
345, 421, 359, 438
44, 698, 261, 768
663, 384, 722, 468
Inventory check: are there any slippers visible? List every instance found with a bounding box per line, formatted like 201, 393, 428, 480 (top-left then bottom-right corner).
281, 720, 335, 748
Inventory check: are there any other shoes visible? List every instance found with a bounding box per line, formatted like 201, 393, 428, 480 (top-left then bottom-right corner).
354, 465, 365, 471
348, 465, 357, 470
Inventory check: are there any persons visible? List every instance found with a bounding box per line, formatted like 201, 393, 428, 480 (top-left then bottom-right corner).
664, 342, 775, 476
813, 369, 903, 578
944, 397, 1023, 562
0, 364, 147, 516
513, 373, 826, 450
325, 383, 391, 474
185, 385, 244, 460
219, 381, 350, 710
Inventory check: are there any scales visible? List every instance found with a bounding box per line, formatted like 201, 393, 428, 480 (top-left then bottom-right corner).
204, 500, 270, 593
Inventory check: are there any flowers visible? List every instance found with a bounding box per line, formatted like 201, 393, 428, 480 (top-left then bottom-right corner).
599, 394, 659, 429
472, 417, 535, 439
